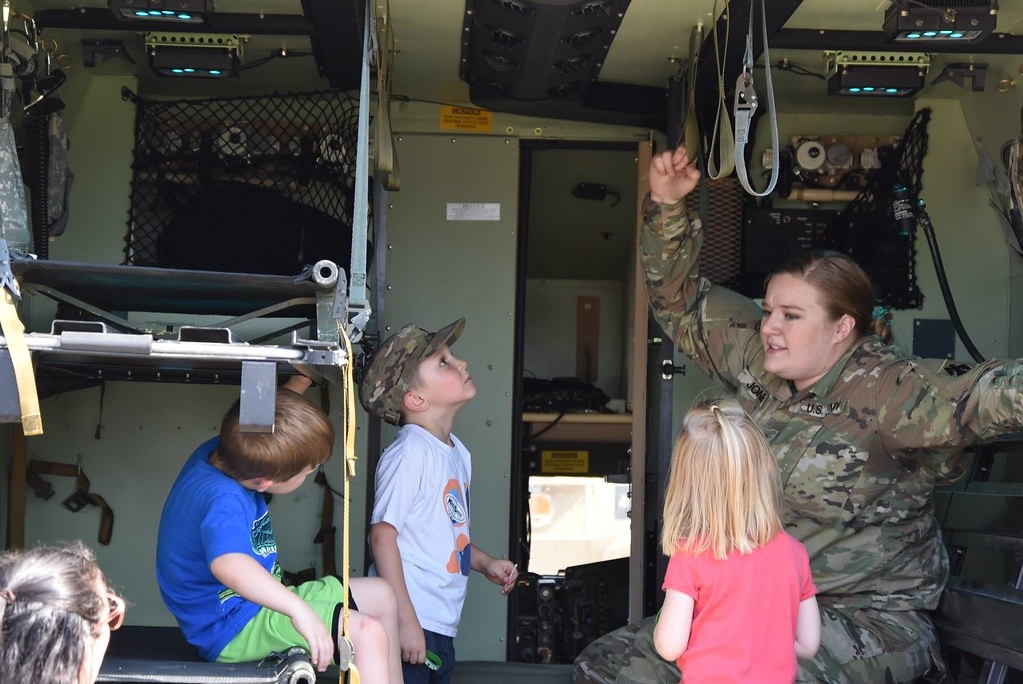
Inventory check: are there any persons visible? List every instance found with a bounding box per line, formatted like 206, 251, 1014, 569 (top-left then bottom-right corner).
360, 318, 520, 684
154, 366, 406, 684
573, 142, 1023, 684
0, 540, 125, 684
653, 396, 823, 684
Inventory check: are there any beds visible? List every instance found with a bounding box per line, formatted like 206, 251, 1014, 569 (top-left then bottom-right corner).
0, 0, 399, 684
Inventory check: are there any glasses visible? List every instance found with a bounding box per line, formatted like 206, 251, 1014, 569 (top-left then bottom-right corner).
88, 595, 126, 638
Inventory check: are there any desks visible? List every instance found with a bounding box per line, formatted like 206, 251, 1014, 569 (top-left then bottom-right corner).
521, 410, 634, 443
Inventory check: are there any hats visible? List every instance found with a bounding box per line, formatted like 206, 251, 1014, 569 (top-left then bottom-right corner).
359, 316, 467, 427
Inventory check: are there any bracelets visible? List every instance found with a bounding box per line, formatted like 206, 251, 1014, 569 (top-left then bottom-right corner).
655, 607, 661, 624
425, 650, 443, 669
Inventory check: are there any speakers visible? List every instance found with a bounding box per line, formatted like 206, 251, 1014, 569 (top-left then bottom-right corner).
537, 579, 558, 664
515, 616, 539, 667
